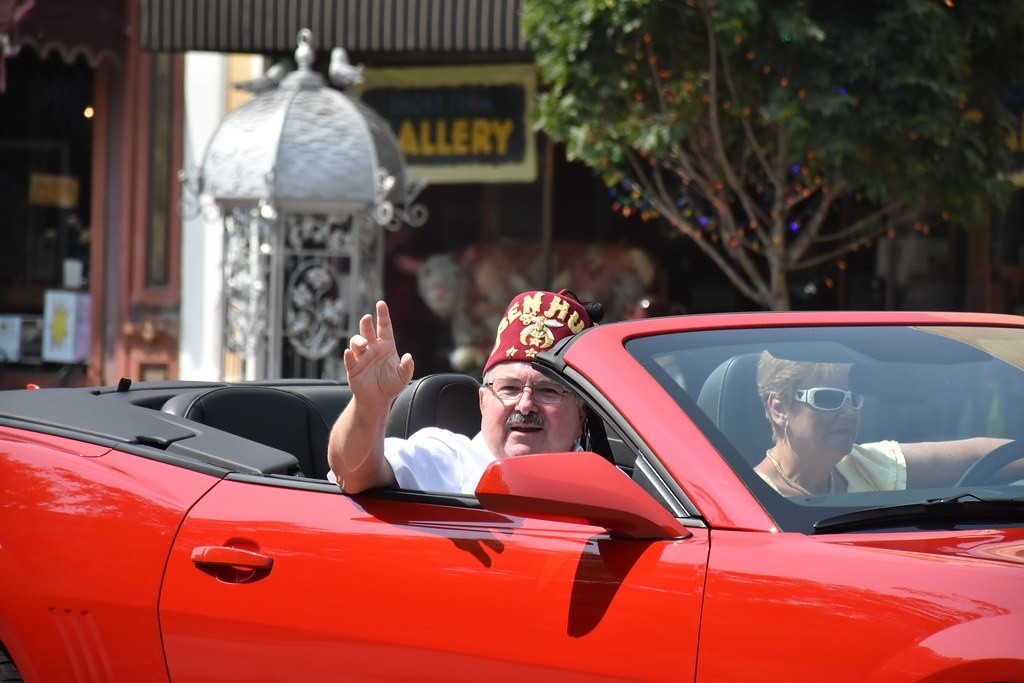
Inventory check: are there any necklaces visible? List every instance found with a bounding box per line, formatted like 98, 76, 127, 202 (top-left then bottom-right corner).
766, 449, 835, 496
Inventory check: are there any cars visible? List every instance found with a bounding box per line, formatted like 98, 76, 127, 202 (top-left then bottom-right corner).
0, 311, 1024, 683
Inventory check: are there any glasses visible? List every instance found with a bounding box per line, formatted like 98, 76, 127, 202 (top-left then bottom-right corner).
475, 377, 566, 405
792, 387, 867, 410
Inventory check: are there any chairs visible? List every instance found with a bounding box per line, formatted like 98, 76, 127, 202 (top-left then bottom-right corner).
385, 372, 481, 439
160, 385, 332, 482
696, 352, 777, 465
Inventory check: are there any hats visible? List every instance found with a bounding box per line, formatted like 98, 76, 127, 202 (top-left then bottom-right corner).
482, 289, 592, 378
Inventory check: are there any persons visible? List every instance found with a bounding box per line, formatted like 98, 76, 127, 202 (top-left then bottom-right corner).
327, 289, 617, 494
753, 350, 1024, 506
